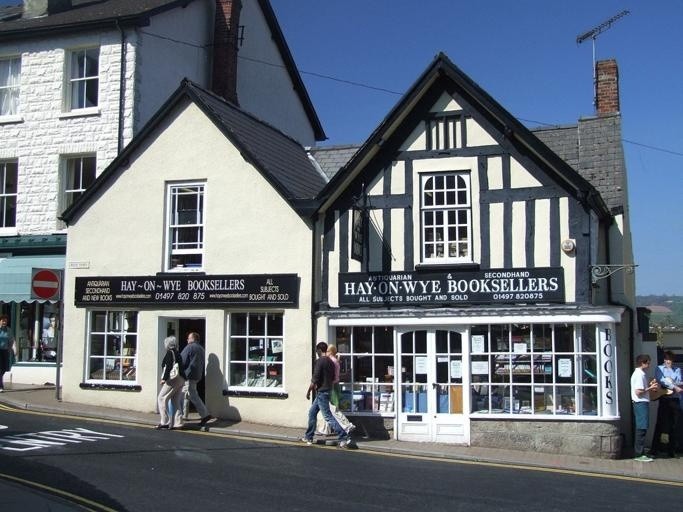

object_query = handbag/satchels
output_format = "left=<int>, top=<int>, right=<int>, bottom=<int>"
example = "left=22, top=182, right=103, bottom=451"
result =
left=161, top=362, right=180, bottom=383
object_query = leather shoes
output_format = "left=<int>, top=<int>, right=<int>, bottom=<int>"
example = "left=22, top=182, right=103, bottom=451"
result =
left=155, top=424, right=169, bottom=430
left=198, top=415, right=212, bottom=427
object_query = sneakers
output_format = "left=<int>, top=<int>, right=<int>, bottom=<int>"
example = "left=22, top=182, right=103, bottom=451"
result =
left=634, top=454, right=654, bottom=462
left=314, top=430, right=327, bottom=437
left=301, top=438, right=312, bottom=444
left=339, top=438, right=351, bottom=448
left=347, top=427, right=359, bottom=439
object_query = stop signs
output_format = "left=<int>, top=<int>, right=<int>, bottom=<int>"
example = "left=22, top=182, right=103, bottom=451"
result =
left=29, top=268, right=63, bottom=300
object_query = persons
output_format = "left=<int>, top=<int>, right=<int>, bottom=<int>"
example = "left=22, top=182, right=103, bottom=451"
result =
left=0, top=313, right=16, bottom=392
left=42, top=313, right=56, bottom=356
left=155, top=335, right=186, bottom=430
left=180, top=331, right=211, bottom=424
left=630, top=354, right=658, bottom=462
left=314, top=344, right=356, bottom=436
left=302, top=342, right=351, bottom=448
left=646, top=353, right=683, bottom=458
